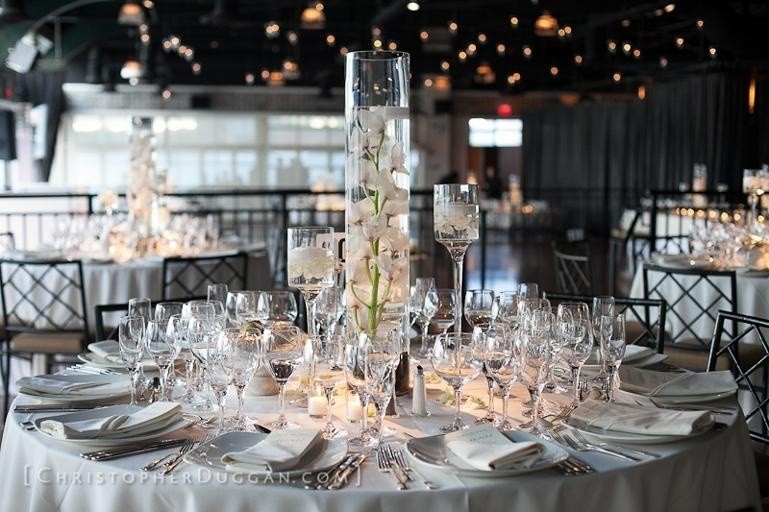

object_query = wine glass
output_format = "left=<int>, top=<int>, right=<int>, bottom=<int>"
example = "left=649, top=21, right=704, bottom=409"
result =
left=118, top=182, right=626, bottom=430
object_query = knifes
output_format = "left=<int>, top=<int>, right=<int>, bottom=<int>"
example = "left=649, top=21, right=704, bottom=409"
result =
left=80, top=437, right=192, bottom=463
left=303, top=450, right=369, bottom=492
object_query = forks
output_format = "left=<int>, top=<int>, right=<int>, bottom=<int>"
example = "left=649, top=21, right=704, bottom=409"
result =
left=373, top=445, right=440, bottom=494
left=137, top=429, right=219, bottom=478
left=547, top=425, right=664, bottom=464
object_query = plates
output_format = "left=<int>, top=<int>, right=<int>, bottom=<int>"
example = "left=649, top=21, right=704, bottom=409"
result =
left=565, top=399, right=715, bottom=443
left=32, top=403, right=202, bottom=445
left=78, top=348, right=189, bottom=370
left=579, top=346, right=669, bottom=373
left=405, top=433, right=573, bottom=476
left=607, top=369, right=739, bottom=401
left=657, top=256, right=714, bottom=270
left=18, top=374, right=139, bottom=399
left=188, top=431, right=351, bottom=477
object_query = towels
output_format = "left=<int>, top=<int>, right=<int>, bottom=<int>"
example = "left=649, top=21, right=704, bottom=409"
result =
left=569, top=397, right=717, bottom=437
left=221, top=428, right=324, bottom=476
left=442, top=422, right=548, bottom=471
left=15, top=364, right=112, bottom=394
left=615, top=362, right=739, bottom=396
left=579, top=344, right=656, bottom=364
left=40, top=400, right=183, bottom=439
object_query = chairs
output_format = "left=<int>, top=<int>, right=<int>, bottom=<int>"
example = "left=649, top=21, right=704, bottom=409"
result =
left=642, top=264, right=764, bottom=379
left=0, top=258, right=96, bottom=425
left=162, top=251, right=248, bottom=302
left=530, top=185, right=667, bottom=354
left=704, top=309, right=768, bottom=446
left=94, top=294, right=207, bottom=340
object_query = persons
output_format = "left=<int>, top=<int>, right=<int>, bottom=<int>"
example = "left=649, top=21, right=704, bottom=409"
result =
left=479, top=164, right=504, bottom=200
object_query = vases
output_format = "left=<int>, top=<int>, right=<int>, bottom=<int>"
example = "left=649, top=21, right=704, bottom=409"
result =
left=128, top=116, right=157, bottom=239
left=342, top=50, right=412, bottom=415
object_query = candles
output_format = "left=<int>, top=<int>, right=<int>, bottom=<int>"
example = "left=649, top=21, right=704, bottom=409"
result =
left=348, top=401, right=363, bottom=420
left=309, top=396, right=326, bottom=416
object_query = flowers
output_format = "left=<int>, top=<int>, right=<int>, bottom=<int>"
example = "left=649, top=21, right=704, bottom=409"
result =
left=345, top=106, right=412, bottom=344
left=130, top=132, right=156, bottom=208
left=433, top=206, right=480, bottom=241
left=288, top=246, right=338, bottom=280
